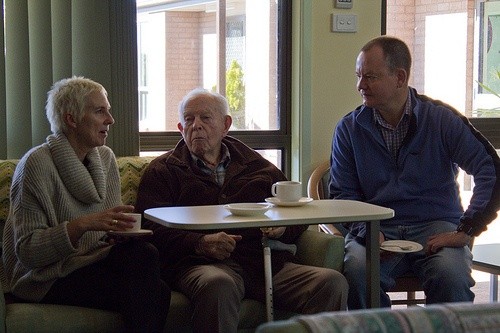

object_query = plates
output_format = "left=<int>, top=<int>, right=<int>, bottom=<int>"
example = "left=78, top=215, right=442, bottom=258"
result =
left=379, top=240, right=424, bottom=252
left=110, top=229, right=153, bottom=235
left=224, top=203, right=273, bottom=216
left=264, top=197, right=313, bottom=206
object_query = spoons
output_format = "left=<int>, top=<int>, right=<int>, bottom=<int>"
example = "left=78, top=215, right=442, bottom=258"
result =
left=381, top=245, right=413, bottom=250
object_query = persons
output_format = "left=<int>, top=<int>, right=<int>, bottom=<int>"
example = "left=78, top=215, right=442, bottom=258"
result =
left=133, top=87, right=349, bottom=333
left=328, top=36, right=500, bottom=312
left=2, top=75, right=173, bottom=333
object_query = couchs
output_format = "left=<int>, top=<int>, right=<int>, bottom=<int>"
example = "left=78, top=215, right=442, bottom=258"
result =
left=0, top=156, right=346, bottom=333
left=255, top=302, right=500, bottom=333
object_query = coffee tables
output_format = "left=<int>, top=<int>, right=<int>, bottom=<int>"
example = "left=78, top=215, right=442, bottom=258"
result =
left=144, top=200, right=394, bottom=325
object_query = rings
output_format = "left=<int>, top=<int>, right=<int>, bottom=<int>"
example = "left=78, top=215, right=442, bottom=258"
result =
left=112, top=220, right=118, bottom=225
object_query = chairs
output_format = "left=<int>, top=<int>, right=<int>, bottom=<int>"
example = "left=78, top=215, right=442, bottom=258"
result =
left=307, top=159, right=428, bottom=308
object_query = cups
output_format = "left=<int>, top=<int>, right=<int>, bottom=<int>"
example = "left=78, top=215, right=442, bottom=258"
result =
left=271, top=181, right=302, bottom=202
left=122, top=213, right=141, bottom=230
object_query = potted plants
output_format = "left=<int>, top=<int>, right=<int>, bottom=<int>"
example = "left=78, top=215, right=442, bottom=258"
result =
left=211, top=59, right=245, bottom=129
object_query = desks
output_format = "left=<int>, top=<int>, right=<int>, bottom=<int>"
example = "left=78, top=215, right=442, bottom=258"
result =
left=470, top=243, right=500, bottom=302
